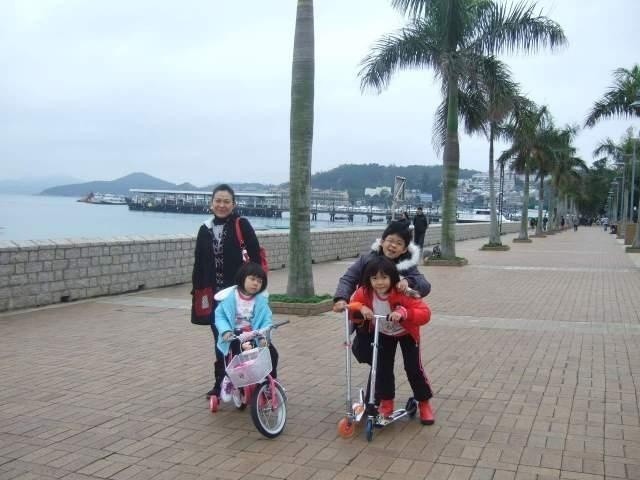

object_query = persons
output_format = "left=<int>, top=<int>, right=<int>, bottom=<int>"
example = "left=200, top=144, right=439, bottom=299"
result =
left=212, top=261, right=280, bottom=401
left=400, top=212, right=412, bottom=230
left=330, top=221, right=432, bottom=419
left=412, top=205, right=428, bottom=251
left=187, top=181, right=270, bottom=404
left=347, top=254, right=437, bottom=428
left=529, top=213, right=610, bottom=232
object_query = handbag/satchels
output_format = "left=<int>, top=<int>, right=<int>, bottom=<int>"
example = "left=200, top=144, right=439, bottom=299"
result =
left=242, top=246, right=268, bottom=273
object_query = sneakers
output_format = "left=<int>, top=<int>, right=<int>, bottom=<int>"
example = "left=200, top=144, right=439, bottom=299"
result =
left=207, top=383, right=221, bottom=398
left=220, top=375, right=233, bottom=403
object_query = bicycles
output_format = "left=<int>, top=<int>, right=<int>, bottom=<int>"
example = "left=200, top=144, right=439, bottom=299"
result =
left=208, top=318, right=291, bottom=439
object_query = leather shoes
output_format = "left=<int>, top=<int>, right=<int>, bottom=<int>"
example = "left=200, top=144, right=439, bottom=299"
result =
left=419, top=400, right=434, bottom=425
left=377, top=400, right=393, bottom=417
